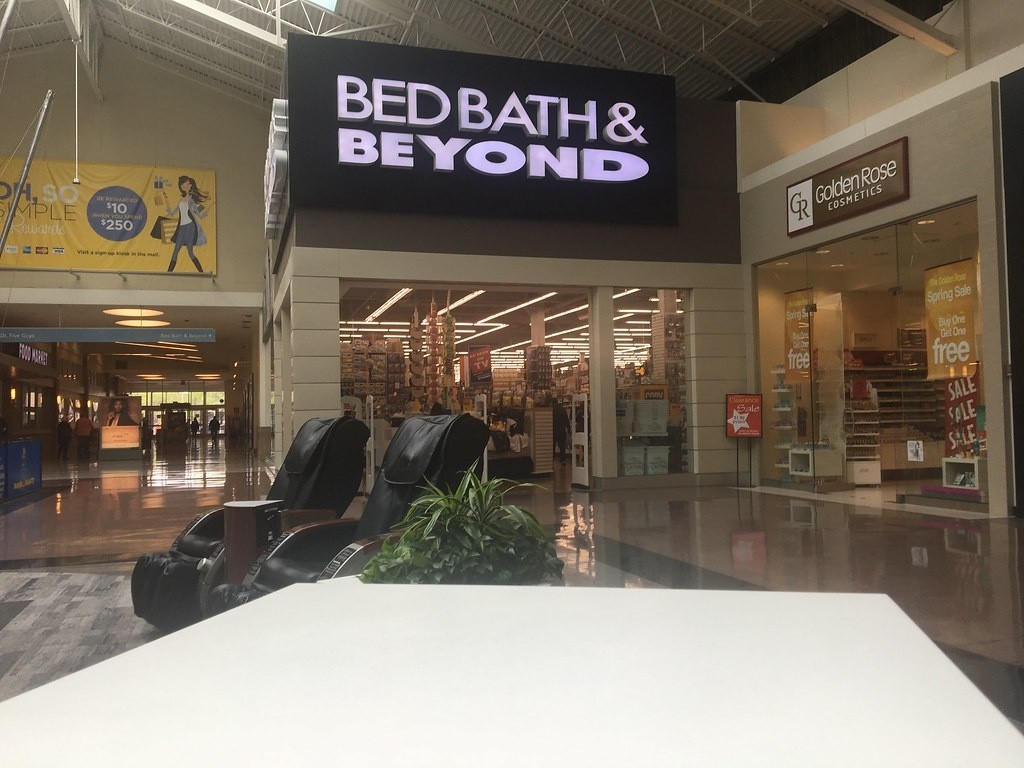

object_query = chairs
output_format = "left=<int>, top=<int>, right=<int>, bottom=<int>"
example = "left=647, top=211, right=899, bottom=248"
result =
left=229, top=413, right=490, bottom=612
left=131, top=416, right=370, bottom=634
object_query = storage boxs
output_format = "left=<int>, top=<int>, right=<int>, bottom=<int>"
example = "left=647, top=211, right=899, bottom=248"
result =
left=615, top=399, right=671, bottom=433
left=618, top=446, right=671, bottom=475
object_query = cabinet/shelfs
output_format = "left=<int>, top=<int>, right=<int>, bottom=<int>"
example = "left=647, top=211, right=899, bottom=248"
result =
left=815, top=365, right=946, bottom=489
left=771, top=369, right=796, bottom=468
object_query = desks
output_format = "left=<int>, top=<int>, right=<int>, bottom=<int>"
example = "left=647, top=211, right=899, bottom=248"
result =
left=617, top=433, right=670, bottom=436
left=2, top=583, right=1024, bottom=765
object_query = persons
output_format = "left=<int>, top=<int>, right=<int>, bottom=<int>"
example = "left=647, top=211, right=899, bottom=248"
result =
left=75, top=411, right=92, bottom=459
left=576, top=401, right=591, bottom=461
left=106, top=397, right=139, bottom=425
left=57, top=416, right=72, bottom=460
left=553, top=398, right=572, bottom=463
left=191, top=417, right=200, bottom=440
left=209, top=416, right=220, bottom=442
